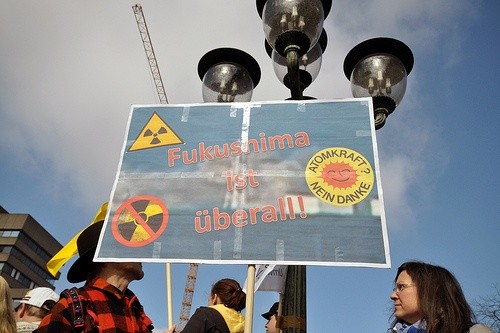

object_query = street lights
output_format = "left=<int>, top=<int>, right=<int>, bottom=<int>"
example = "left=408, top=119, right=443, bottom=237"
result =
left=199, top=0, right=414, bottom=333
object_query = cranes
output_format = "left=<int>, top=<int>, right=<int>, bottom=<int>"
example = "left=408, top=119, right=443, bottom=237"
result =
left=129, top=4, right=198, bottom=332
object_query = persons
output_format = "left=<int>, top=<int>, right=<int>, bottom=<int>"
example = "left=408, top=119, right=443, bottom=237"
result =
left=0, top=275, right=60, bottom=333
left=387, top=261, right=496, bottom=333
left=178, top=278, right=246, bottom=333
left=261, top=302, right=280, bottom=333
left=31, top=219, right=178, bottom=333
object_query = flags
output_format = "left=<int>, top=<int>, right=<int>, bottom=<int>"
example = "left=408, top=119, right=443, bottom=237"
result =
left=242, top=265, right=287, bottom=294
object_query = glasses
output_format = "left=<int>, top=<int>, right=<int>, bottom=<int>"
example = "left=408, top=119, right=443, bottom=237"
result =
left=393, top=282, right=415, bottom=293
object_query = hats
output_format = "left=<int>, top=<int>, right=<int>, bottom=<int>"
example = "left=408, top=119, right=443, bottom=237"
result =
left=67, top=220, right=104, bottom=283
left=261, top=302, right=279, bottom=321
left=20, top=287, right=61, bottom=310
